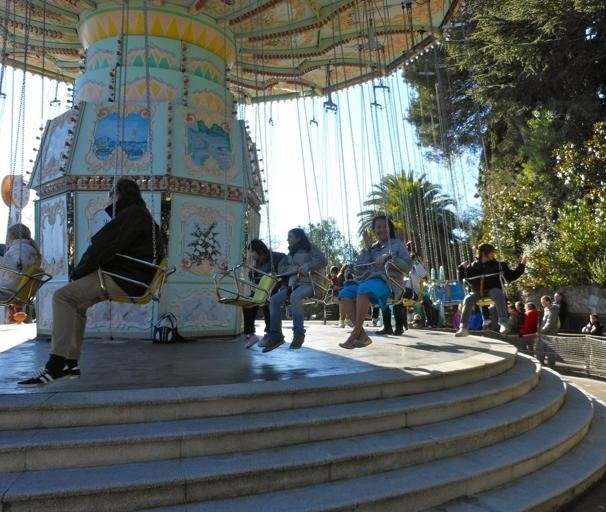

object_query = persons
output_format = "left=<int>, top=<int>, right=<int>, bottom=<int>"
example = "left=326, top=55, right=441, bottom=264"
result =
left=0, top=224, right=44, bottom=303
left=241, top=238, right=287, bottom=348
left=581, top=312, right=601, bottom=377
left=452, top=243, right=530, bottom=339
left=15, top=177, right=168, bottom=387
left=454, top=290, right=567, bottom=371
left=321, top=253, right=432, bottom=336
left=338, top=215, right=415, bottom=350
left=260, top=227, right=330, bottom=355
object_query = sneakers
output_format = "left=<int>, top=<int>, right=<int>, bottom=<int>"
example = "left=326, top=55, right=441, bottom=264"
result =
left=339, top=335, right=373, bottom=349
left=289, top=333, right=306, bottom=350
left=16, top=365, right=81, bottom=388
left=338, top=319, right=354, bottom=328
left=243, top=334, right=258, bottom=349
left=375, top=327, right=393, bottom=335
left=500, top=324, right=512, bottom=334
left=455, top=327, right=470, bottom=337
left=393, top=329, right=404, bottom=336
left=372, top=318, right=378, bottom=327
left=258, top=333, right=286, bottom=353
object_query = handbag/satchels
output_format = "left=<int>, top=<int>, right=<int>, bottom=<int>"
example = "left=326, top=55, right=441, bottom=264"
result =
left=152, top=311, right=179, bottom=344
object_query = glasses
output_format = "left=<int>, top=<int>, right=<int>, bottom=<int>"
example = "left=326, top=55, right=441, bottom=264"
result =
left=108, top=190, right=121, bottom=197
